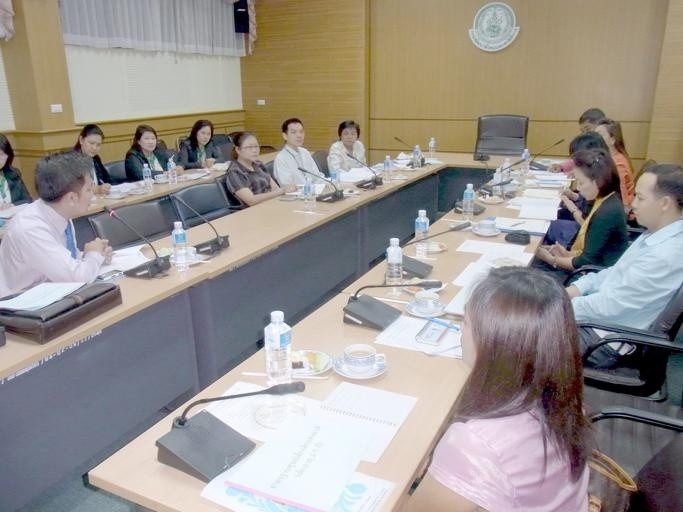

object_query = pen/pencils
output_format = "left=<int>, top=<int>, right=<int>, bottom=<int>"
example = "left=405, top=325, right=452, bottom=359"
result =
left=511, top=222, right=525, bottom=227
left=427, top=315, right=461, bottom=332
left=99, top=177, right=104, bottom=185
left=559, top=186, right=567, bottom=196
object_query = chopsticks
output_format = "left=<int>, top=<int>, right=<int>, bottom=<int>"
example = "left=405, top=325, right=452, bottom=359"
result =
left=293, top=210, right=325, bottom=218
left=240, top=371, right=331, bottom=381
left=350, top=294, right=410, bottom=306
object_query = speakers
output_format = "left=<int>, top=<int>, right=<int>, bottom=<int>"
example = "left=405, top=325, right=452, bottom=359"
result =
left=232, top=1, right=251, bottom=34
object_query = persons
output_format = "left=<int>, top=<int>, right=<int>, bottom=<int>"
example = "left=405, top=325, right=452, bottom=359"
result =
left=388, top=265, right=592, bottom=510
left=536, top=109, right=635, bottom=285
left=225, top=131, right=299, bottom=206
left=325, top=121, right=366, bottom=179
left=72, top=123, right=116, bottom=197
left=272, top=118, right=334, bottom=190
left=0, top=133, right=33, bottom=213
left=124, top=124, right=185, bottom=182
left=563, top=163, right=682, bottom=371
left=177, top=120, right=226, bottom=170
left=0, top=150, right=115, bottom=301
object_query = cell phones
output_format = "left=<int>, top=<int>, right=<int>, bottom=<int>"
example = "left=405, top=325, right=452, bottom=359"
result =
left=98, top=268, right=124, bottom=284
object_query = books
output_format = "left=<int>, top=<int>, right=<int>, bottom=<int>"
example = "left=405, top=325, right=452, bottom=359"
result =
left=494, top=216, right=551, bottom=236
left=414, top=316, right=452, bottom=346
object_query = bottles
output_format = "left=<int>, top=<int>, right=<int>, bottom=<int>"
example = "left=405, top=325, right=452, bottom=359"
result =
left=462, top=184, right=475, bottom=222
left=383, top=155, right=392, bottom=179
left=385, top=238, right=404, bottom=288
left=521, top=149, right=530, bottom=173
left=414, top=209, right=429, bottom=260
left=428, top=138, right=438, bottom=161
left=330, top=166, right=340, bottom=189
left=142, top=163, right=153, bottom=193
left=171, top=220, right=191, bottom=273
left=412, top=145, right=422, bottom=168
left=166, top=158, right=178, bottom=186
left=303, top=178, right=316, bottom=211
left=493, top=168, right=504, bottom=201
left=502, top=158, right=511, bottom=176
left=263, top=309, right=293, bottom=388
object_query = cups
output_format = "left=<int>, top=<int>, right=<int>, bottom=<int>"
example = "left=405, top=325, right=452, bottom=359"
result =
left=414, top=290, right=440, bottom=314
left=345, top=343, right=387, bottom=374
left=473, top=221, right=496, bottom=233
left=172, top=246, right=197, bottom=260
left=155, top=174, right=165, bottom=181
left=109, top=188, right=123, bottom=196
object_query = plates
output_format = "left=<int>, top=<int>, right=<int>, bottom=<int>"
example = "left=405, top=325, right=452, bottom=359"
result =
left=479, top=196, right=504, bottom=205
left=333, top=358, right=389, bottom=381
left=169, top=256, right=202, bottom=265
left=401, top=278, right=446, bottom=295
left=413, top=241, right=446, bottom=253
left=471, top=229, right=501, bottom=237
left=389, top=175, right=409, bottom=180
left=405, top=302, right=449, bottom=319
left=104, top=194, right=125, bottom=199
left=288, top=349, right=334, bottom=375
left=151, top=179, right=170, bottom=184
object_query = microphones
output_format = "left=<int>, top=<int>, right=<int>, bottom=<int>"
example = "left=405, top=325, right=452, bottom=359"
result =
left=156, top=379, right=305, bottom=484
left=100, top=204, right=171, bottom=279
left=342, top=279, right=443, bottom=331
left=346, top=152, right=382, bottom=189
left=502, top=157, right=526, bottom=171
left=394, top=136, right=425, bottom=167
left=298, top=166, right=344, bottom=203
left=401, top=222, right=473, bottom=279
left=530, top=138, right=567, bottom=172
left=169, top=192, right=230, bottom=254
left=455, top=178, right=515, bottom=215
